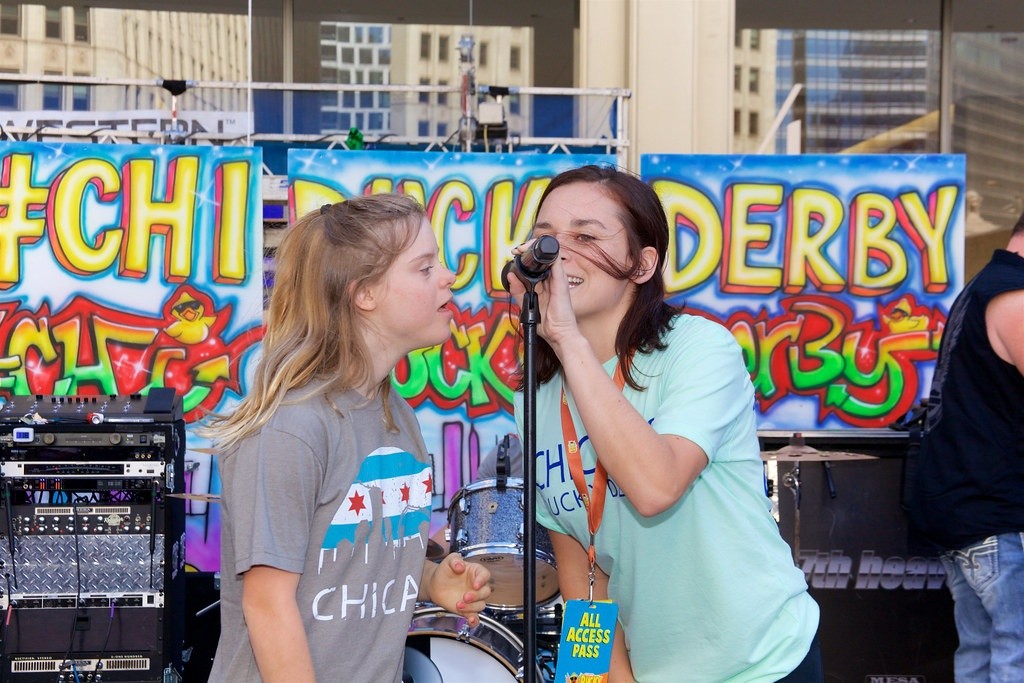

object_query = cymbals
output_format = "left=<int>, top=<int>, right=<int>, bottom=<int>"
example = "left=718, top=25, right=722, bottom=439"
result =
left=761, top=443, right=880, bottom=462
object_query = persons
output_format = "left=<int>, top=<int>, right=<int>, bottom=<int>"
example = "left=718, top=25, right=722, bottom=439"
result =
left=195, top=193, right=491, bottom=683
left=426, top=434, right=524, bottom=558
left=922, top=211, right=1024, bottom=683
left=507, top=165, right=824, bottom=683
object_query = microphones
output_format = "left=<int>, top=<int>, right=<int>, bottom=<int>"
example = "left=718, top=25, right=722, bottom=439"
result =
left=499, top=232, right=559, bottom=292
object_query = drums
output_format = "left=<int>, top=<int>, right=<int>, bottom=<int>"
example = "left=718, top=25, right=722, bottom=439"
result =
left=404, top=606, right=546, bottom=682
left=446, top=476, right=561, bottom=611
